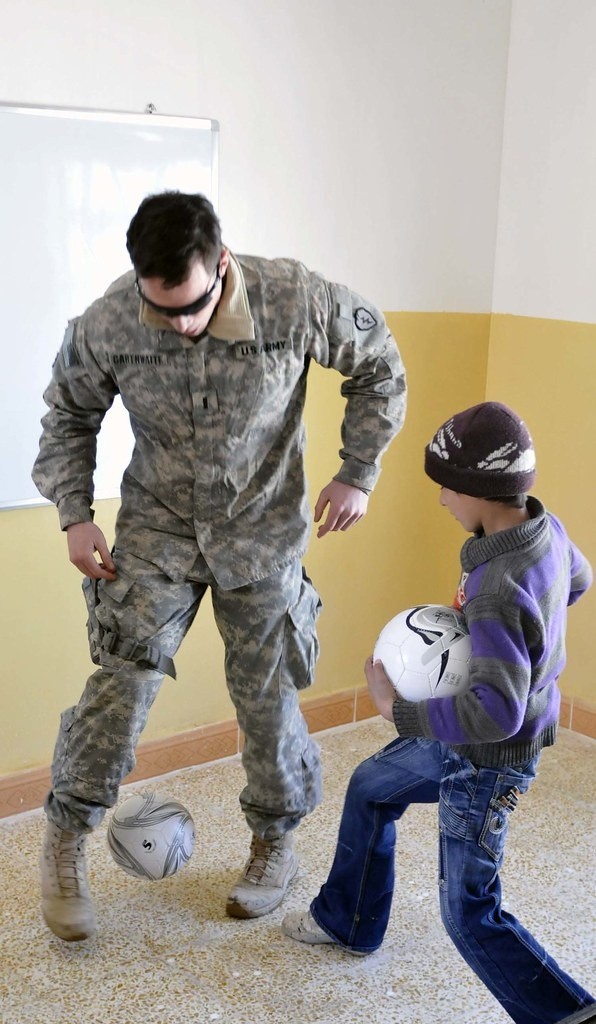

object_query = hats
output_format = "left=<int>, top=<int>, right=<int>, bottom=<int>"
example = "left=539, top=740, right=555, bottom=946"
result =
left=422, top=401, right=536, bottom=498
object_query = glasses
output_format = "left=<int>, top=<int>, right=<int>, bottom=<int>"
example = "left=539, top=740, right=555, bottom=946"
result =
left=134, top=261, right=220, bottom=318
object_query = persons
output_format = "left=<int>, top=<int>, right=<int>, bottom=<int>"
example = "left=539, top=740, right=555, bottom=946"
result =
left=30, top=189, right=407, bottom=941
left=280, top=401, right=595, bottom=1023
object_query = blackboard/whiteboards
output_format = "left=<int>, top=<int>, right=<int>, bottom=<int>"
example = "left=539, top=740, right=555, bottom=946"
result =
left=0, top=100, right=220, bottom=512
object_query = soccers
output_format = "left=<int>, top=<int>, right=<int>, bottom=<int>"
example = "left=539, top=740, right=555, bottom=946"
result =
left=107, top=792, right=196, bottom=881
left=373, top=603, right=473, bottom=703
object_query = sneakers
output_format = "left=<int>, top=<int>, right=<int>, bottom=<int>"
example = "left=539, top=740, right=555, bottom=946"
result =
left=281, top=908, right=381, bottom=957
left=224, top=831, right=298, bottom=918
left=556, top=1002, right=596, bottom=1024
left=38, top=819, right=97, bottom=943
